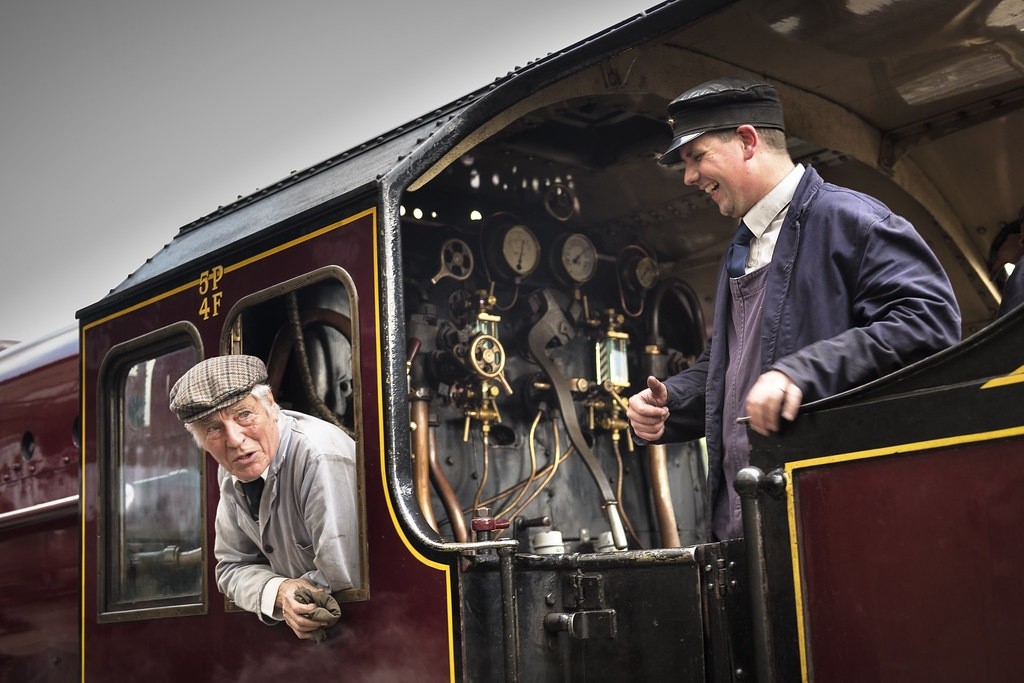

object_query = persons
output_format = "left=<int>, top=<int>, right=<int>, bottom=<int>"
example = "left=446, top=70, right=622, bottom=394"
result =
left=627, top=78, right=962, bottom=546
left=168, top=354, right=362, bottom=640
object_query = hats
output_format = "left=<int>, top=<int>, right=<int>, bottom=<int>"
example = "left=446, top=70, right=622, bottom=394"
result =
left=168, top=355, right=269, bottom=424
left=658, top=78, right=785, bottom=165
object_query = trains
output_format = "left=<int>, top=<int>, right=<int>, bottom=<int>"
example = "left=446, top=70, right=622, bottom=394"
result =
left=0, top=0, right=1024, bottom=683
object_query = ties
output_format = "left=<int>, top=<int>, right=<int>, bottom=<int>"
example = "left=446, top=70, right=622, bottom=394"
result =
left=242, top=477, right=266, bottom=518
left=728, top=224, right=753, bottom=279
